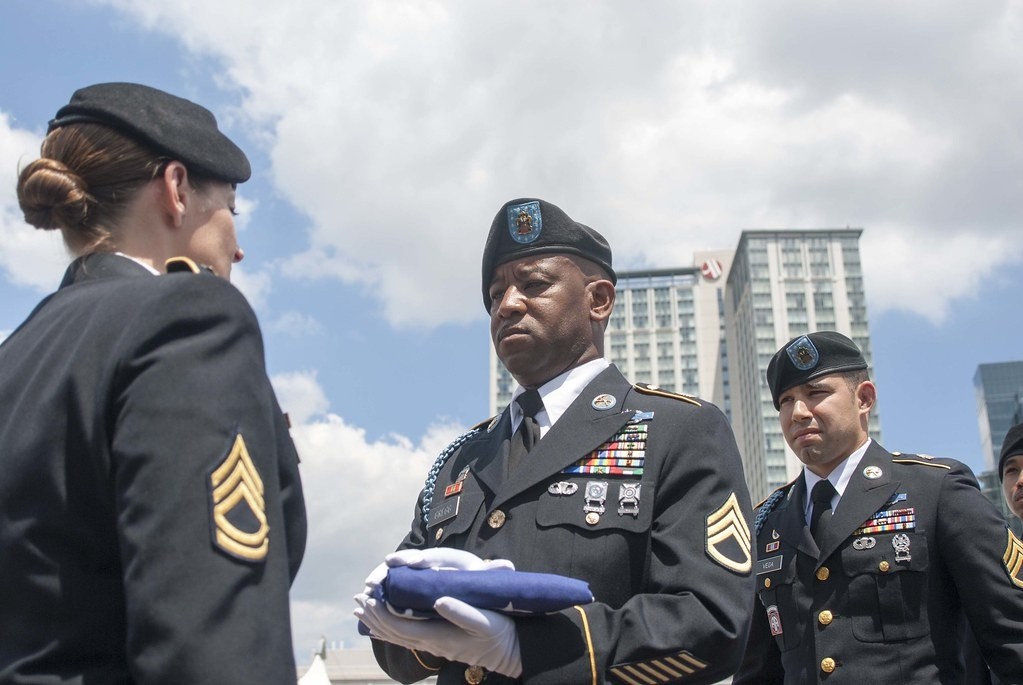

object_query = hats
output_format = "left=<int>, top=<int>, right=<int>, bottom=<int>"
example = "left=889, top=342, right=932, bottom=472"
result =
left=482, top=198, right=618, bottom=321
left=998, top=423, right=1023, bottom=484
left=767, top=332, right=868, bottom=411
left=48, top=82, right=252, bottom=183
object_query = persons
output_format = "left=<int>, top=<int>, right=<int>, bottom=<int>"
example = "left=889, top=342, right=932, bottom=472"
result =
left=353, top=197, right=756, bottom=685
left=997, top=423, right=1023, bottom=517
left=732, top=332, right=1023, bottom=685
left=0, top=82, right=307, bottom=685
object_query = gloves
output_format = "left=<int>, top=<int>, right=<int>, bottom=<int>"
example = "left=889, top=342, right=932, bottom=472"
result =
left=351, top=594, right=523, bottom=679
left=362, top=547, right=516, bottom=595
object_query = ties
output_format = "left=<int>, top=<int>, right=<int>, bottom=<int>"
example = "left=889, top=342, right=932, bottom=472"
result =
left=507, top=391, right=544, bottom=482
left=810, top=480, right=837, bottom=551
